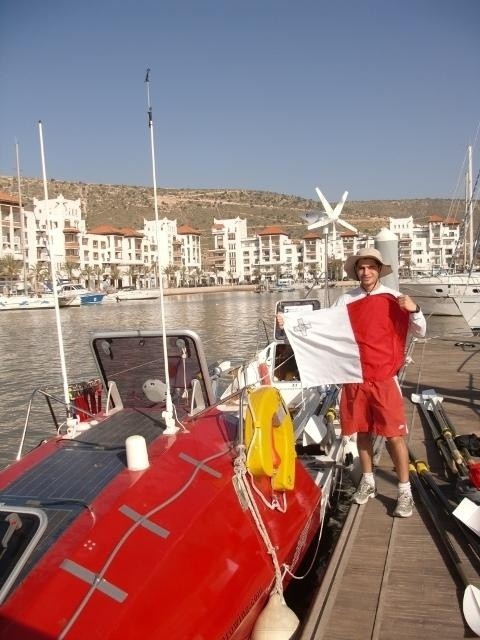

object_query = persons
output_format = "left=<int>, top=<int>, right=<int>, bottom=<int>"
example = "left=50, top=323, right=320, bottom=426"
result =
left=276, top=247, right=426, bottom=518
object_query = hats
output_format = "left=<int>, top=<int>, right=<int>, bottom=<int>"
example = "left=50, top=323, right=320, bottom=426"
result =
left=344, top=246, right=394, bottom=281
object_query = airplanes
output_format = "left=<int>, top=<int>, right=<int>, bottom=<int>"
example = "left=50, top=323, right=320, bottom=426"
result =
left=0, top=180, right=359, bottom=640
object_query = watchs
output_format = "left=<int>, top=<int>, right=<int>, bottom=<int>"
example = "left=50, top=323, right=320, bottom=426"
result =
left=410, top=305, right=420, bottom=313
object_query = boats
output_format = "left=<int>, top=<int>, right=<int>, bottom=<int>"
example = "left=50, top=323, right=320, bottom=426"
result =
left=267, top=278, right=336, bottom=292
left=0, top=143, right=162, bottom=310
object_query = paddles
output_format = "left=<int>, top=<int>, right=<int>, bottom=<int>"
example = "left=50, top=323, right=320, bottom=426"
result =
left=384, top=389, right=480, bottom=636
left=303, top=383, right=341, bottom=456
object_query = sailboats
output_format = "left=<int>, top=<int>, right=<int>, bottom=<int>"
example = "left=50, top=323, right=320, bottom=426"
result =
left=394, top=139, right=479, bottom=330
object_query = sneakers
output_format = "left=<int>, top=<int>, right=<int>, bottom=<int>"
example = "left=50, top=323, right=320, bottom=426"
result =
left=391, top=492, right=416, bottom=518
left=349, top=479, right=377, bottom=506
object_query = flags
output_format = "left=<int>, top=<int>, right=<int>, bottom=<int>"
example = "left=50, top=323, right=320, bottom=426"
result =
left=278, top=293, right=410, bottom=390
